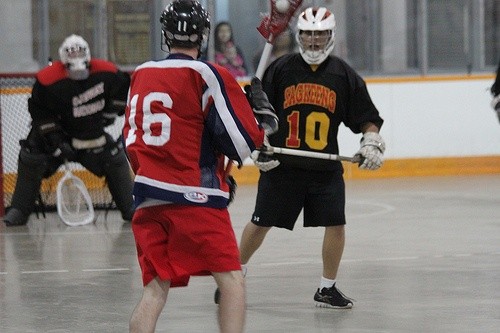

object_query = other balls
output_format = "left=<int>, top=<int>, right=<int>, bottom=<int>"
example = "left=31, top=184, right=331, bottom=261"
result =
left=275, top=0, right=289, bottom=13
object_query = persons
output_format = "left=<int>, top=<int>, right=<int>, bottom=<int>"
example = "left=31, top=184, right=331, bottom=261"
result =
left=120, top=0, right=278, bottom=333
left=215, top=7, right=386, bottom=310
left=2, top=34, right=135, bottom=226
left=214, top=22, right=248, bottom=78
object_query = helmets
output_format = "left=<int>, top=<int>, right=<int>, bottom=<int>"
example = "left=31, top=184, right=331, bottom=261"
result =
left=58, top=33, right=90, bottom=71
left=160, top=0, right=210, bottom=46
left=295, top=6, right=336, bottom=65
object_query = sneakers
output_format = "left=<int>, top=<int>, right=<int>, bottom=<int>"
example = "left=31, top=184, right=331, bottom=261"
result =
left=214, top=288, right=221, bottom=304
left=313, top=282, right=357, bottom=310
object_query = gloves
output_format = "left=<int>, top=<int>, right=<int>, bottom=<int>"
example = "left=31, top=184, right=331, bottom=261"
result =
left=249, top=135, right=280, bottom=172
left=244, top=78, right=277, bottom=119
left=92, top=134, right=119, bottom=156
left=351, top=131, right=386, bottom=171
left=38, top=128, right=68, bottom=164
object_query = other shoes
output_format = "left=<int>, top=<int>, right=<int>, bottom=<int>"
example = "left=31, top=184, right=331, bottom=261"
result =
left=121, top=201, right=136, bottom=221
left=3, top=206, right=28, bottom=226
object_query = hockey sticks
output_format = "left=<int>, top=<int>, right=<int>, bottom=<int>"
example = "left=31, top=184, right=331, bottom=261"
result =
left=223, top=0, right=303, bottom=183
left=254, top=144, right=353, bottom=162
left=56, top=158, right=95, bottom=226
